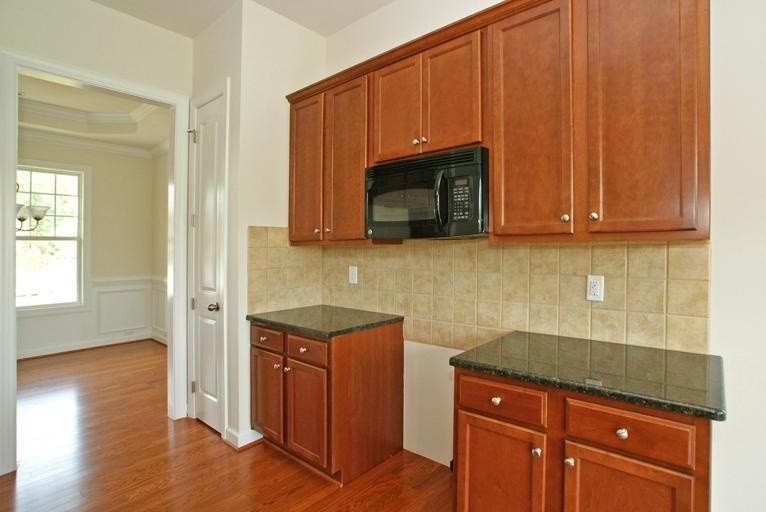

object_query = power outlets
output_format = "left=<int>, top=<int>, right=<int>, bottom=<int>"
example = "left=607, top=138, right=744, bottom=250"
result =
left=587, top=275, right=604, bottom=302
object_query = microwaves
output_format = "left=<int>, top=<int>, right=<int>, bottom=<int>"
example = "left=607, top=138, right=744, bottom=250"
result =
left=365, top=146, right=489, bottom=239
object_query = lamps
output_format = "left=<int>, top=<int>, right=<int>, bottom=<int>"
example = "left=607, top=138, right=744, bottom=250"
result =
left=17, top=183, right=50, bottom=231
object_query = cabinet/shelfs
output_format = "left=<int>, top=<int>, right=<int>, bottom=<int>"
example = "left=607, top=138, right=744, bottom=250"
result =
left=453, top=366, right=710, bottom=512
left=483, top=0, right=710, bottom=245
left=368, top=10, right=483, bottom=165
left=286, top=60, right=368, bottom=245
left=250, top=321, right=403, bottom=489
